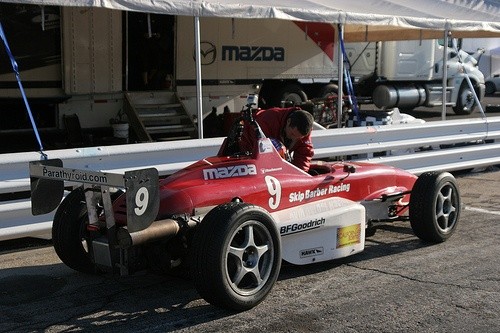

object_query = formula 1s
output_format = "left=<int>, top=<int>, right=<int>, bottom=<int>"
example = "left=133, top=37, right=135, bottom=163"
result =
left=29, top=103, right=461, bottom=309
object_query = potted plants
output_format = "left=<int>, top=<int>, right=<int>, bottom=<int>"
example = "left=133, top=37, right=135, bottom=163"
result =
left=108, top=109, right=130, bottom=138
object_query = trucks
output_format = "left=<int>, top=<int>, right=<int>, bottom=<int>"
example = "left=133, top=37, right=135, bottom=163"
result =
left=61, top=7, right=485, bottom=138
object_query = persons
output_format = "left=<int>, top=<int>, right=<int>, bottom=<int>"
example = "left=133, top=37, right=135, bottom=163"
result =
left=218, top=106, right=315, bottom=172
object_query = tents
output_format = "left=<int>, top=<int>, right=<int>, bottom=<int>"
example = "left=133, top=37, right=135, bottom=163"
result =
left=1, top=0, right=500, bottom=139
